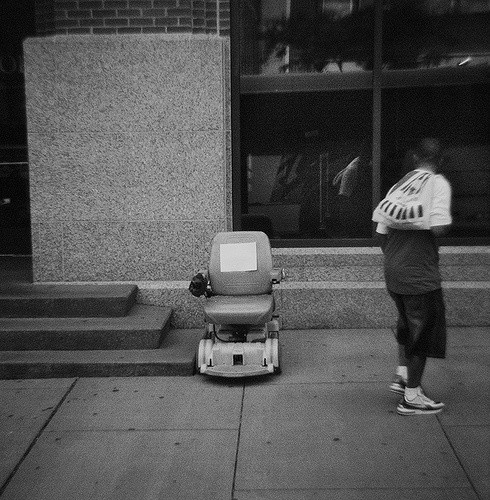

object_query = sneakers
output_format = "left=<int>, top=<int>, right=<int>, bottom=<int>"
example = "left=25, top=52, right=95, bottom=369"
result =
left=396, top=394, right=445, bottom=416
left=389, top=375, right=426, bottom=396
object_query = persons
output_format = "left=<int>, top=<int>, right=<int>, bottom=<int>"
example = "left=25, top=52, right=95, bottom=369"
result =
left=371, top=135, right=452, bottom=415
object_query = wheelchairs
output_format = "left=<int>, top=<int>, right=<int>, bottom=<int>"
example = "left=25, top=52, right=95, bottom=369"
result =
left=188, top=231, right=282, bottom=377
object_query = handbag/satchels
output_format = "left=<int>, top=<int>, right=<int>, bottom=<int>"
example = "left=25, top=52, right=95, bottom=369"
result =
left=332, top=168, right=346, bottom=185
left=371, top=171, right=435, bottom=231
